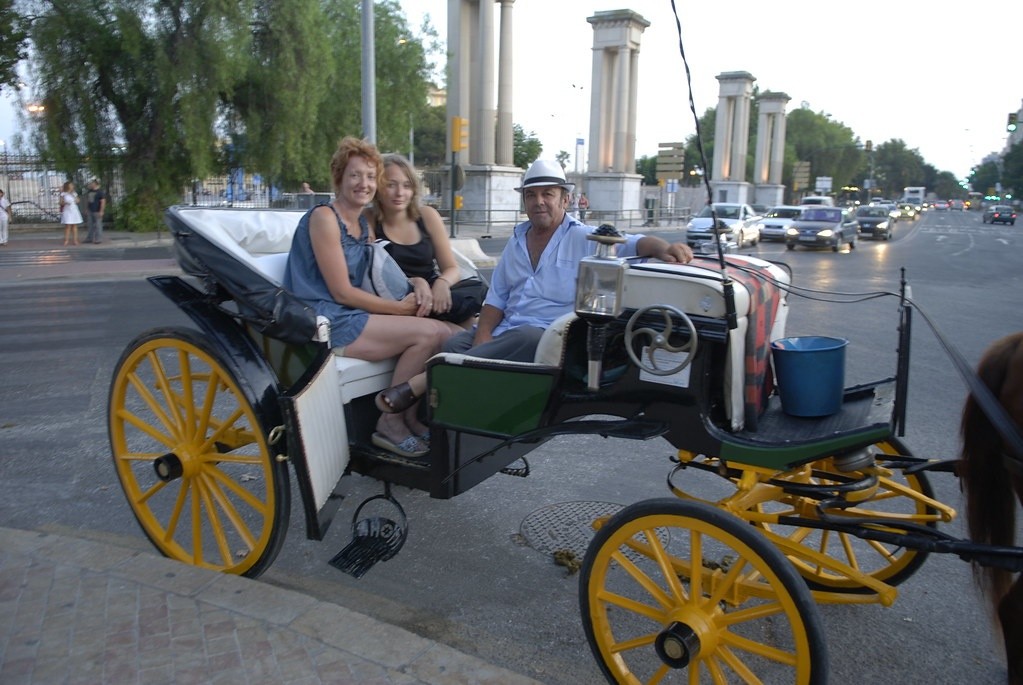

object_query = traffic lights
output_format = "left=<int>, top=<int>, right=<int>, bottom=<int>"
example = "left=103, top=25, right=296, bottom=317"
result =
left=451, top=116, right=469, bottom=152
left=455, top=195, right=463, bottom=208
left=1007, top=113, right=1017, bottom=132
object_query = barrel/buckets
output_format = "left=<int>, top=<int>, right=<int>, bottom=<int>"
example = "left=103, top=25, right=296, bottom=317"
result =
left=773, top=335, right=849, bottom=419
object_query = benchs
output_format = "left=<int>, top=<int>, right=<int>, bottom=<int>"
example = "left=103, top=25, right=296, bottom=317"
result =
left=166, top=204, right=488, bottom=399
left=426, top=310, right=582, bottom=437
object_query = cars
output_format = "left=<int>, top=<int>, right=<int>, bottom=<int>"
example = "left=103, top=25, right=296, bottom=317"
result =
left=686, top=202, right=760, bottom=251
left=760, top=196, right=923, bottom=252
left=923, top=199, right=964, bottom=211
left=983, top=205, right=1017, bottom=226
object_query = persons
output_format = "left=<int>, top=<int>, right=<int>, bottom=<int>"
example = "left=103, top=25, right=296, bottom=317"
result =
left=283, top=136, right=453, bottom=457
left=60, top=182, right=83, bottom=246
left=81, top=176, right=106, bottom=243
left=578, top=192, right=589, bottom=224
left=0, top=189, right=11, bottom=246
left=360, top=155, right=490, bottom=335
left=303, top=183, right=313, bottom=193
left=375, top=156, right=693, bottom=414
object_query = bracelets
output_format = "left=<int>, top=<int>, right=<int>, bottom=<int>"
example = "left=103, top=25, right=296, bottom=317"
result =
left=438, top=277, right=450, bottom=285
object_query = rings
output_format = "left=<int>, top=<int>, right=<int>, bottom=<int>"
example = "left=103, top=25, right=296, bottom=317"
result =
left=429, top=304, right=433, bottom=306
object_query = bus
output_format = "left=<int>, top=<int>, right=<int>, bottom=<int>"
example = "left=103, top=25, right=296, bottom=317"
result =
left=904, top=187, right=926, bottom=208
left=967, top=192, right=983, bottom=209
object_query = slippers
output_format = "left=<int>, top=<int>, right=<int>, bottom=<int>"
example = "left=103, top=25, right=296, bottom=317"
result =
left=372, top=432, right=430, bottom=457
left=412, top=428, right=433, bottom=447
left=374, top=382, right=427, bottom=414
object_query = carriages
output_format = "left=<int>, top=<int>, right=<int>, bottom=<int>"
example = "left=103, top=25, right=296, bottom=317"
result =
left=106, top=204, right=1023, bottom=685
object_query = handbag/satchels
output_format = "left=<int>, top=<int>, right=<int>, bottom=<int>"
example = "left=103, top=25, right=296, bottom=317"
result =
left=424, top=278, right=488, bottom=324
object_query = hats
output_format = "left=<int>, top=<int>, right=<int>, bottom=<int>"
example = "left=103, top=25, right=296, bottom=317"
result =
left=513, top=158, right=575, bottom=193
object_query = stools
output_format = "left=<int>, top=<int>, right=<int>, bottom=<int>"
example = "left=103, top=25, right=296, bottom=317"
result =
left=619, top=248, right=792, bottom=431
